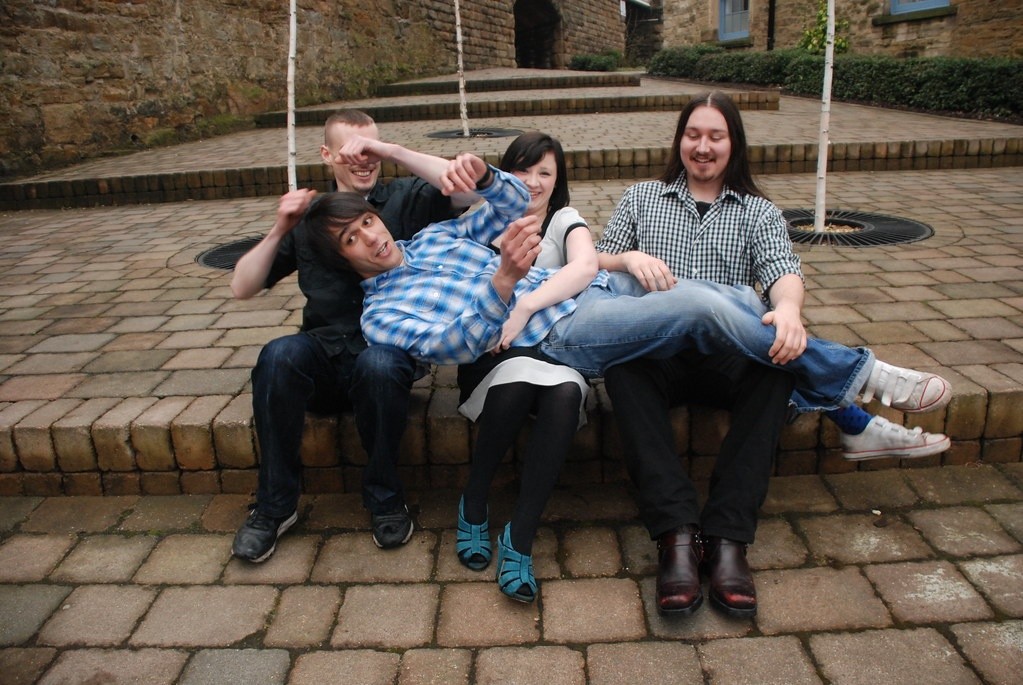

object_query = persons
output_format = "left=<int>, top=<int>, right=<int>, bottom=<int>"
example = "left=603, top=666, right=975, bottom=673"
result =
left=230, top=110, right=481, bottom=563
left=304, top=153, right=952, bottom=461
left=457, top=132, right=599, bottom=605
left=594, top=91, right=807, bottom=617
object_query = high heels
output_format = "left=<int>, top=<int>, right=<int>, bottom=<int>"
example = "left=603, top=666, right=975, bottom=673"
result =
left=456, top=491, right=494, bottom=571
left=494, top=522, right=537, bottom=603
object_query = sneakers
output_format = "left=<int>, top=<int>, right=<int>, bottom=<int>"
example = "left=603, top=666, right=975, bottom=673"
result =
left=839, top=414, right=951, bottom=462
left=231, top=503, right=296, bottom=562
left=858, top=359, right=953, bottom=413
left=372, top=503, right=414, bottom=550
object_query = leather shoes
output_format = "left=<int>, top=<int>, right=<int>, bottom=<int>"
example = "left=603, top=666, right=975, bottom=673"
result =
left=656, top=523, right=703, bottom=617
left=697, top=529, right=757, bottom=617
